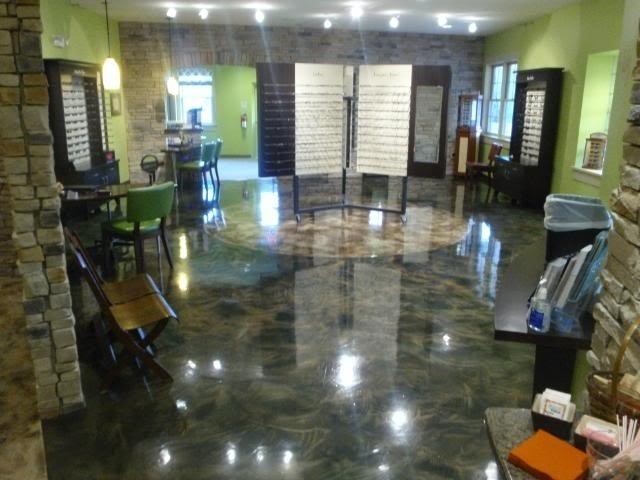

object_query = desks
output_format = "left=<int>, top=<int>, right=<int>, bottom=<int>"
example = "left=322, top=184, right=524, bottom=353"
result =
left=494, top=239, right=593, bottom=404
left=63, top=183, right=151, bottom=263
left=482, top=407, right=590, bottom=479
left=161, top=143, right=203, bottom=189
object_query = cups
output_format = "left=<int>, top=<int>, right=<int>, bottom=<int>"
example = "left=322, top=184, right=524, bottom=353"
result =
left=585, top=431, right=640, bottom=480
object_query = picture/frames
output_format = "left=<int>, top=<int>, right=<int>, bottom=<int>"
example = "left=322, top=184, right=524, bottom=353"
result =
left=110, top=93, right=122, bottom=116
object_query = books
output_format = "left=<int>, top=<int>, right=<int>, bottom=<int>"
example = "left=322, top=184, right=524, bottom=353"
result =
left=506, top=427, right=594, bottom=480
left=526, top=228, right=608, bottom=320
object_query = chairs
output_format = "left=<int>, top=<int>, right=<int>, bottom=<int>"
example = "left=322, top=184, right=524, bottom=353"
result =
left=175, top=141, right=215, bottom=192
left=464, top=142, right=502, bottom=190
left=100, top=180, right=174, bottom=277
left=191, top=139, right=223, bottom=188
left=62, top=225, right=181, bottom=394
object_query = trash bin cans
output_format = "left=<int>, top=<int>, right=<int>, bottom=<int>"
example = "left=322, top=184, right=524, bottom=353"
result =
left=544, top=194, right=611, bottom=269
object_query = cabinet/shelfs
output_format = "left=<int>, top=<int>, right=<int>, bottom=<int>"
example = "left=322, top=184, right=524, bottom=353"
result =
left=45, top=59, right=121, bottom=221
left=493, top=66, right=565, bottom=203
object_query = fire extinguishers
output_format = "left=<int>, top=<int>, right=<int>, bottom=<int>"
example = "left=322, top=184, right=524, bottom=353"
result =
left=241, top=113, right=247, bottom=128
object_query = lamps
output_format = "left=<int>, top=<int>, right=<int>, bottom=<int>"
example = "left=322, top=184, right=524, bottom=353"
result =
left=166, top=18, right=178, bottom=97
left=102, top=0, right=121, bottom=90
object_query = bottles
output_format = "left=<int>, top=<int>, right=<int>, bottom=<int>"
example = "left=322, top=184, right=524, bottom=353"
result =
left=528, top=278, right=551, bottom=333
